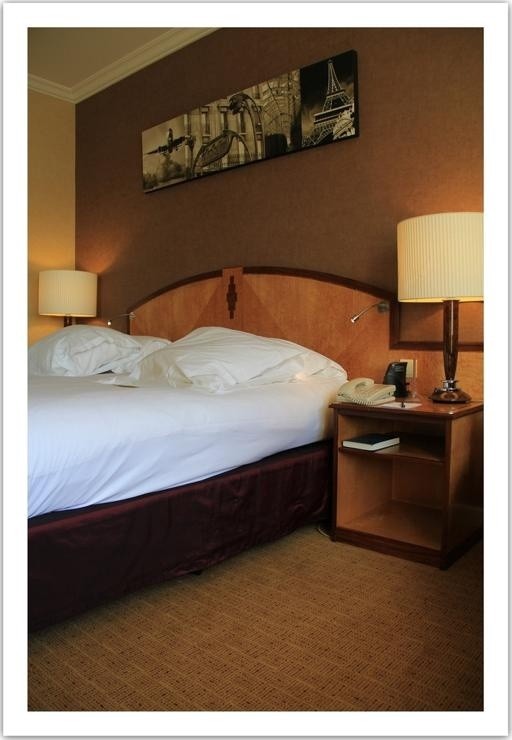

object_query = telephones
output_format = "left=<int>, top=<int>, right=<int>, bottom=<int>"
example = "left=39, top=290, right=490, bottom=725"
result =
left=336, top=377, right=396, bottom=405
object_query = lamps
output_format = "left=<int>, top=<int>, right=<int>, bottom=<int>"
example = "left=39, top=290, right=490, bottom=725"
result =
left=396, top=211, right=484, bottom=406
left=107, top=313, right=136, bottom=326
left=350, top=301, right=389, bottom=325
left=39, top=270, right=98, bottom=327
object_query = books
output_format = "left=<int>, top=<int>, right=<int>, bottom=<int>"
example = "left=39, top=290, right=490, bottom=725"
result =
left=343, top=433, right=400, bottom=451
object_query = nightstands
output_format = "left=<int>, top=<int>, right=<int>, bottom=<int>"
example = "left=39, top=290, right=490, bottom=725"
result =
left=329, top=397, right=484, bottom=571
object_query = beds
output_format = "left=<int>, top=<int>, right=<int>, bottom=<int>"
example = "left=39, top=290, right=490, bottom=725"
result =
left=27, top=267, right=399, bottom=634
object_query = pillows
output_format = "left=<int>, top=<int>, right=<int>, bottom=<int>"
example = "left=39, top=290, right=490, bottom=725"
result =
left=28, top=324, right=172, bottom=376
left=100, top=325, right=348, bottom=391
